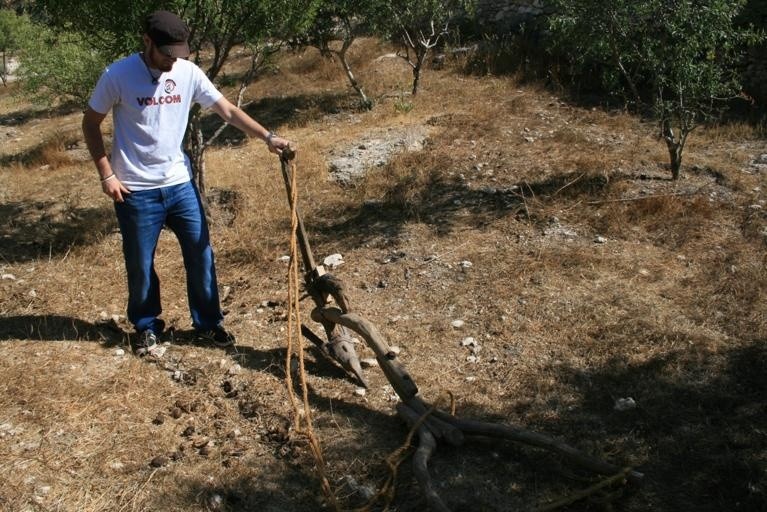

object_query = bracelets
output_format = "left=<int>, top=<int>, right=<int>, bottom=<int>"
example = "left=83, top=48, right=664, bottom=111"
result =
left=98, top=173, right=115, bottom=182
left=264, top=128, right=274, bottom=142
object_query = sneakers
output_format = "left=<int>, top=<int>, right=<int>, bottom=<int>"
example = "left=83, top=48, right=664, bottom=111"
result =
left=136, top=331, right=160, bottom=353
left=200, top=324, right=236, bottom=346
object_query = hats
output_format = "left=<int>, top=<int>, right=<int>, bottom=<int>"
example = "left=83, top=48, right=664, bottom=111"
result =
left=143, top=11, right=190, bottom=58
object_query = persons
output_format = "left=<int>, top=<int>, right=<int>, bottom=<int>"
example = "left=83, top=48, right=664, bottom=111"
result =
left=82, top=10, right=297, bottom=354
